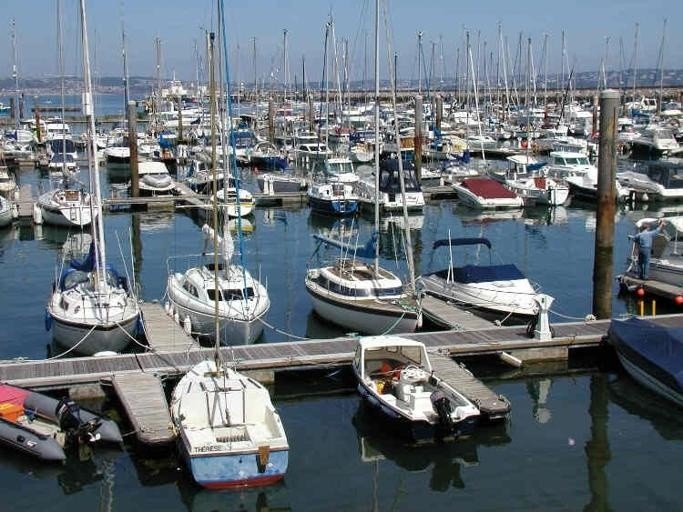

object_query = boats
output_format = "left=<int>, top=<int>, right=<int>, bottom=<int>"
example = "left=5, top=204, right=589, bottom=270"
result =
left=604, top=376, right=683, bottom=441
left=1, top=377, right=125, bottom=468
left=603, top=310, right=683, bottom=407
left=346, top=330, right=482, bottom=449
left=349, top=398, right=480, bottom=492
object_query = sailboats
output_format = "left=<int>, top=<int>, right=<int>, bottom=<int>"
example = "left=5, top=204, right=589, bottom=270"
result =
left=166, top=20, right=291, bottom=493
left=170, top=467, right=292, bottom=511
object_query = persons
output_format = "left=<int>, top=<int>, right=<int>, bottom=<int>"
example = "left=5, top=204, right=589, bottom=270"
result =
left=626, top=219, right=664, bottom=281
left=429, top=389, right=461, bottom=441
